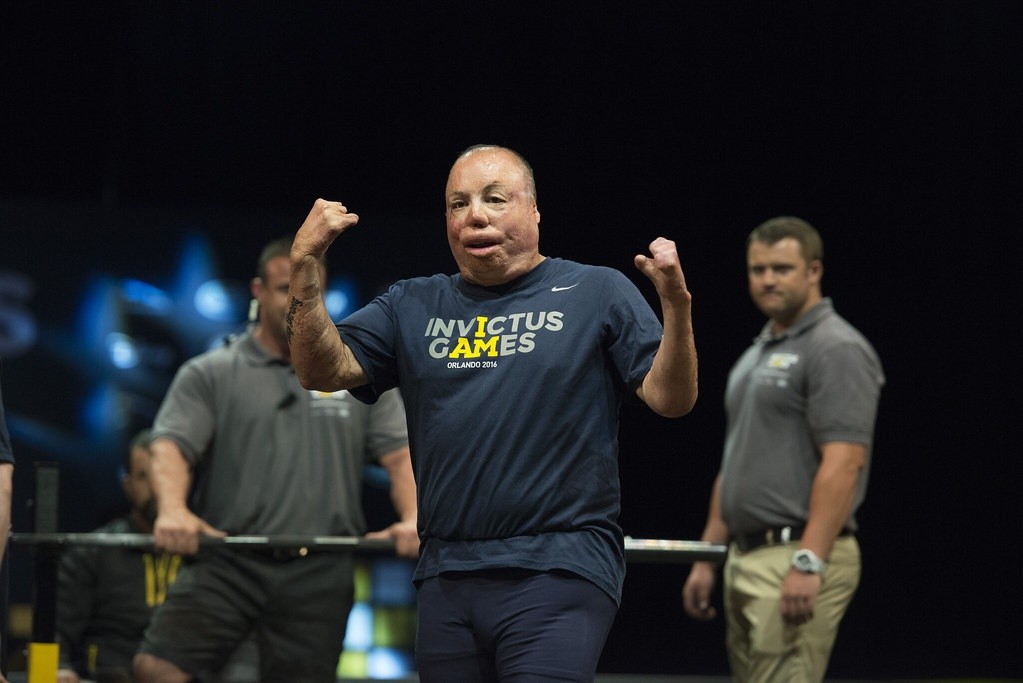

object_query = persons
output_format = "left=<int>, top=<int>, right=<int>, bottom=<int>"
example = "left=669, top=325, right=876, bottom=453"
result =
left=682, top=215, right=884, bottom=683
left=282, top=144, right=700, bottom=683
left=58, top=430, right=186, bottom=683
left=131, top=238, right=422, bottom=683
left=0, top=394, right=16, bottom=566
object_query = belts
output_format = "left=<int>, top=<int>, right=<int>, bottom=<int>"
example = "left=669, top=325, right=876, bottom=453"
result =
left=730, top=523, right=851, bottom=553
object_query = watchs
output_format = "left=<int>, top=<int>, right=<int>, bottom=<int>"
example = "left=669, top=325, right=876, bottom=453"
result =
left=790, top=548, right=828, bottom=574
